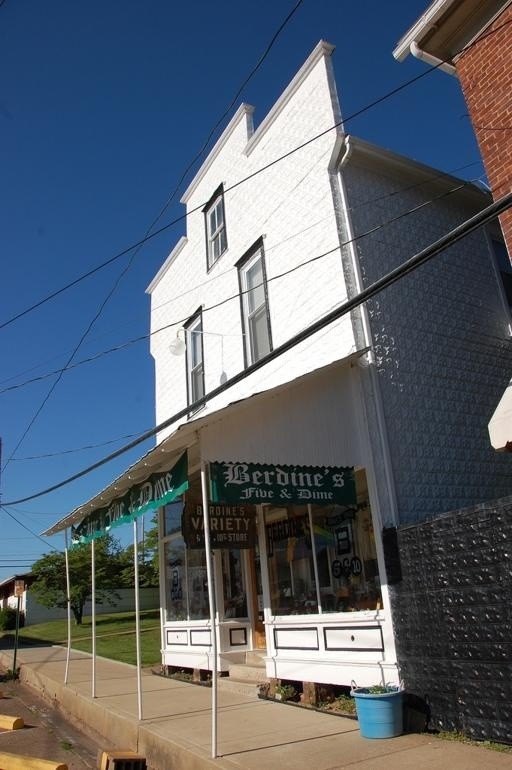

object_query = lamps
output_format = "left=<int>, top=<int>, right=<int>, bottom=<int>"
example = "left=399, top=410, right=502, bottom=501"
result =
left=168, top=327, right=225, bottom=357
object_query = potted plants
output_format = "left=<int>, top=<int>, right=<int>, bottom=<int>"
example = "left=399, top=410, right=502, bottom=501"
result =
left=349, top=679, right=406, bottom=739
